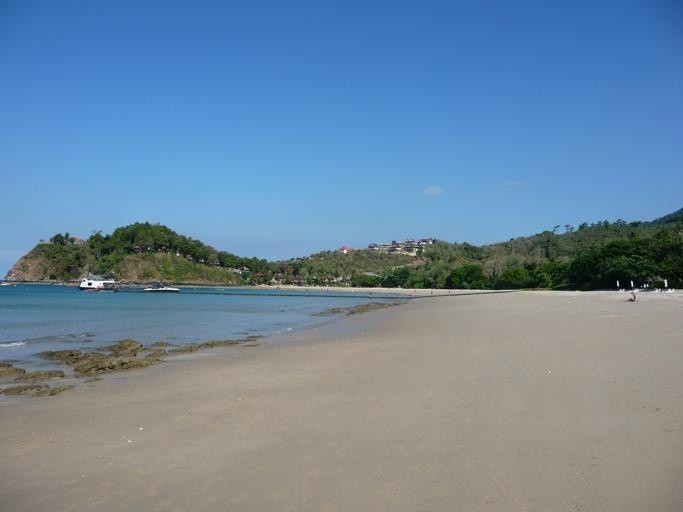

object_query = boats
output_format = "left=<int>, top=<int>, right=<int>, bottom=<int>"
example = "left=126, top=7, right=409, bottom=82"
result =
left=142, top=281, right=180, bottom=293
left=77, top=274, right=117, bottom=290
